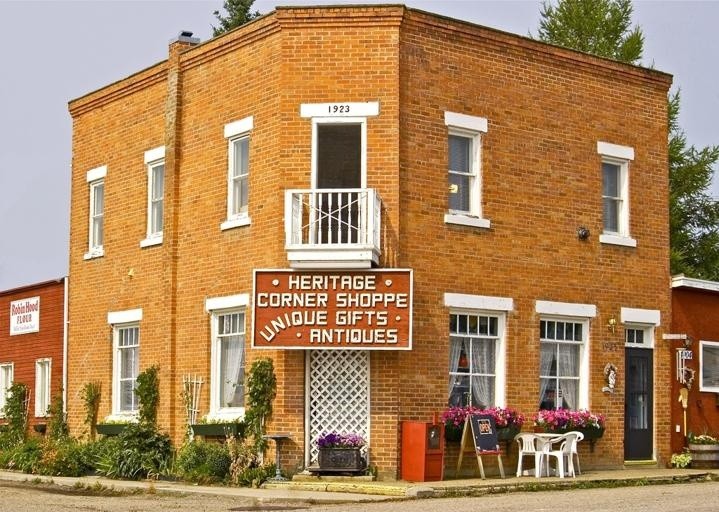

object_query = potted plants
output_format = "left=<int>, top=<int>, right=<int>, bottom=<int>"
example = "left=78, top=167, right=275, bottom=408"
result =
left=689, top=443, right=719, bottom=469
left=191, top=416, right=246, bottom=436
left=95, top=419, right=140, bottom=436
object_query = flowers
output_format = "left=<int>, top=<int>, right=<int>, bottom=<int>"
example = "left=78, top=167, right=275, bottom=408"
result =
left=671, top=452, right=692, bottom=469
left=442, top=406, right=605, bottom=432
left=318, top=433, right=366, bottom=448
left=687, top=433, right=718, bottom=445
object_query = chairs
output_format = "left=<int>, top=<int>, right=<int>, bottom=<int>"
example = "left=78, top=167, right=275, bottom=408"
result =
left=512, top=430, right=585, bottom=478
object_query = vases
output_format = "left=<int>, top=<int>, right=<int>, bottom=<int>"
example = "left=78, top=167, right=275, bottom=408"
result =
left=318, top=447, right=361, bottom=469
